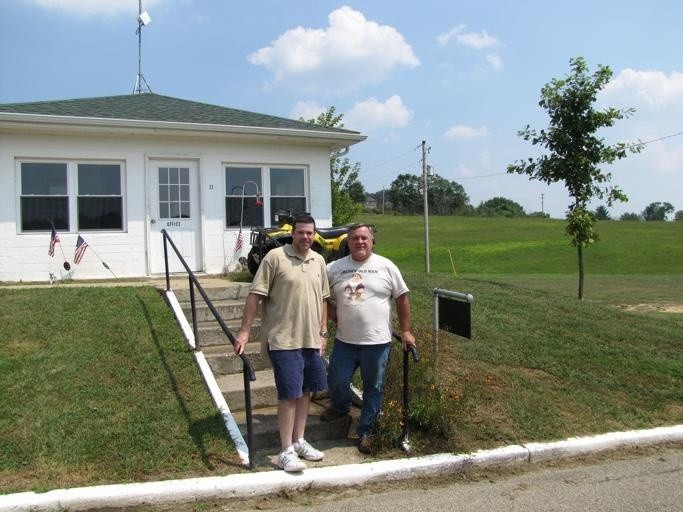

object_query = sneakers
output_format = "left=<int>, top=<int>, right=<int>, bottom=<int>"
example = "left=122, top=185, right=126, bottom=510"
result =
left=293, top=440, right=325, bottom=461
left=276, top=446, right=306, bottom=472
left=359, top=438, right=371, bottom=452
left=320, top=407, right=350, bottom=421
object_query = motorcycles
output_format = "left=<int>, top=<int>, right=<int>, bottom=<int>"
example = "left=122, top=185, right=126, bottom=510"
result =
left=247, top=209, right=377, bottom=277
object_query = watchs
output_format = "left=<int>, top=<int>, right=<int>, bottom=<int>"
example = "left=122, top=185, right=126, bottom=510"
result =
left=318, top=331, right=330, bottom=338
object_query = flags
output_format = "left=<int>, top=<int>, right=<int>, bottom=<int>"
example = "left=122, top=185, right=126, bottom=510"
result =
left=72, top=235, right=88, bottom=265
left=235, top=227, right=245, bottom=254
left=46, top=224, right=60, bottom=258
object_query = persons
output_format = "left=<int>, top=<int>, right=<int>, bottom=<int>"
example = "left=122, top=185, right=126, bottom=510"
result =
left=230, top=212, right=332, bottom=474
left=320, top=222, right=418, bottom=454
